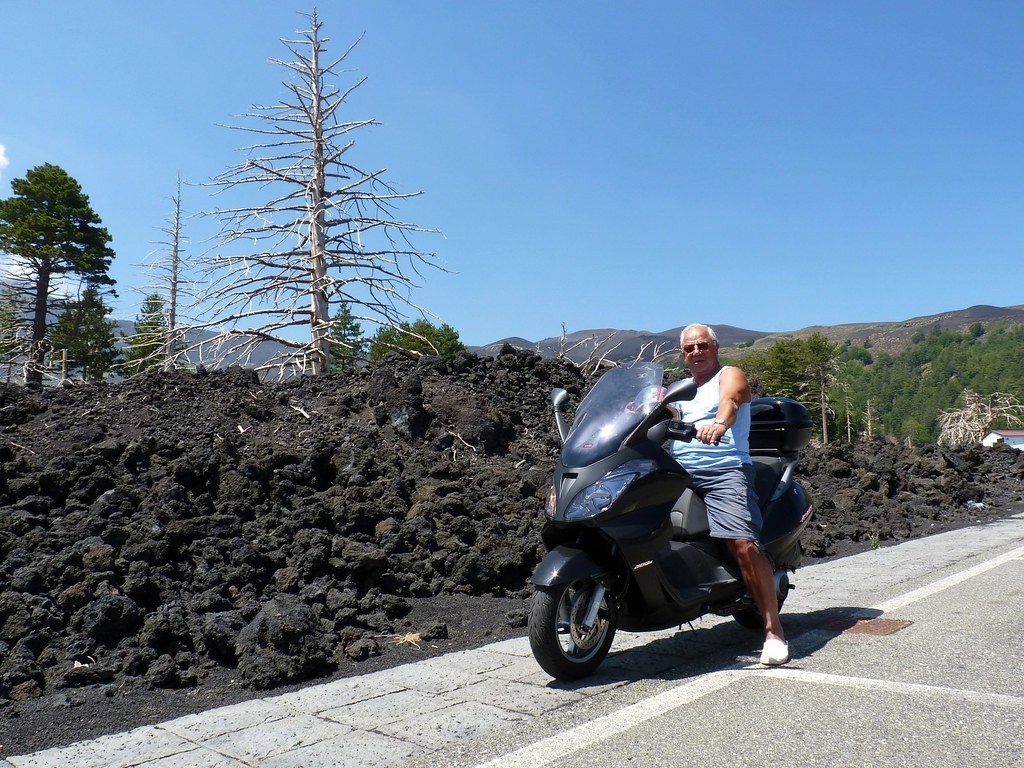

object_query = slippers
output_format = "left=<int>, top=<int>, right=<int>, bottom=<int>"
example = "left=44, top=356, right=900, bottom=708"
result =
left=759, top=640, right=789, bottom=664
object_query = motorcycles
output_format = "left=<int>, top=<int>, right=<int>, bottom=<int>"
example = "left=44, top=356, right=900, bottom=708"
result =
left=525, top=360, right=816, bottom=681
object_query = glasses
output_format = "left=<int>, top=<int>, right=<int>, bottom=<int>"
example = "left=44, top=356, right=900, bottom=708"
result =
left=684, top=342, right=712, bottom=353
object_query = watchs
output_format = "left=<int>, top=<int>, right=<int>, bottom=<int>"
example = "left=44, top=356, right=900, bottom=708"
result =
left=712, top=417, right=728, bottom=428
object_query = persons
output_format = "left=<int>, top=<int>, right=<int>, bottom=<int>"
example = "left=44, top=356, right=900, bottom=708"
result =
left=622, top=324, right=790, bottom=665
